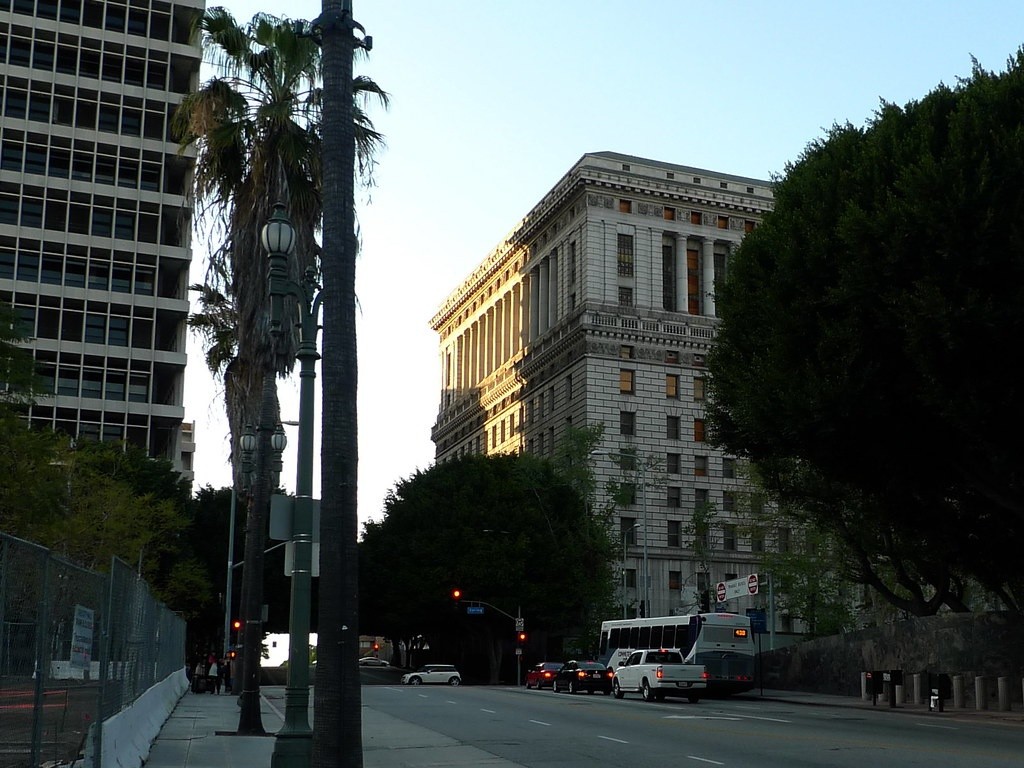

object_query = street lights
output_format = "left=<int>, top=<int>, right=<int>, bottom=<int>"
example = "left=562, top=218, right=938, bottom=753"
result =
left=258, top=205, right=326, bottom=768
left=591, top=450, right=649, bottom=618
left=220, top=420, right=301, bottom=695
left=231, top=421, right=287, bottom=697
left=622, top=523, right=644, bottom=618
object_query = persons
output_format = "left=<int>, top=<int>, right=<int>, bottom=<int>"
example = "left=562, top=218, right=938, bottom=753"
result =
left=207, top=649, right=237, bottom=694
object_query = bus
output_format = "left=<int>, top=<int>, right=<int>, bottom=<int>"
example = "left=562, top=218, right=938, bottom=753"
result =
left=598, top=612, right=756, bottom=699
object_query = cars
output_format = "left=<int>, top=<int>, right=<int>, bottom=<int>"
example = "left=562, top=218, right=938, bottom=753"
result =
left=400, top=664, right=462, bottom=688
left=525, top=662, right=564, bottom=690
left=553, top=660, right=612, bottom=695
left=359, top=656, right=390, bottom=668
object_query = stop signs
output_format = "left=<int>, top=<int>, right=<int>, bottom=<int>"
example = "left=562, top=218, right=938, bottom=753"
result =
left=717, top=583, right=726, bottom=601
left=748, top=575, right=758, bottom=594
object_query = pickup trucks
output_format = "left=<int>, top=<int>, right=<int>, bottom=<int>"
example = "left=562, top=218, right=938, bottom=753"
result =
left=611, top=647, right=708, bottom=703
